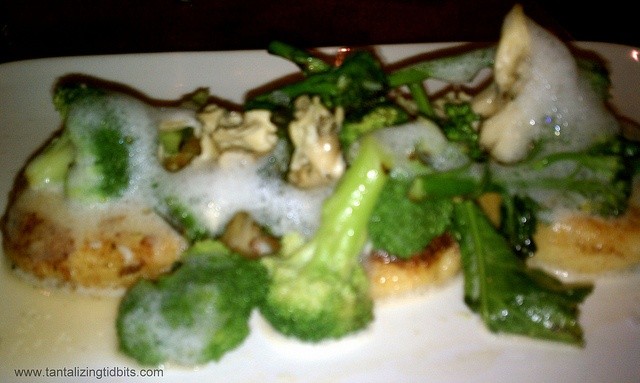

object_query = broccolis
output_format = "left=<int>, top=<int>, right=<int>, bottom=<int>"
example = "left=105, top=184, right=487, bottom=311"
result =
left=260, top=139, right=390, bottom=343
left=115, top=236, right=268, bottom=367
left=369, top=179, right=456, bottom=263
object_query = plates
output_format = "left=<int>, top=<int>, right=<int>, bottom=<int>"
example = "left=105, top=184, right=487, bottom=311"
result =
left=1, top=41, right=636, bottom=382
